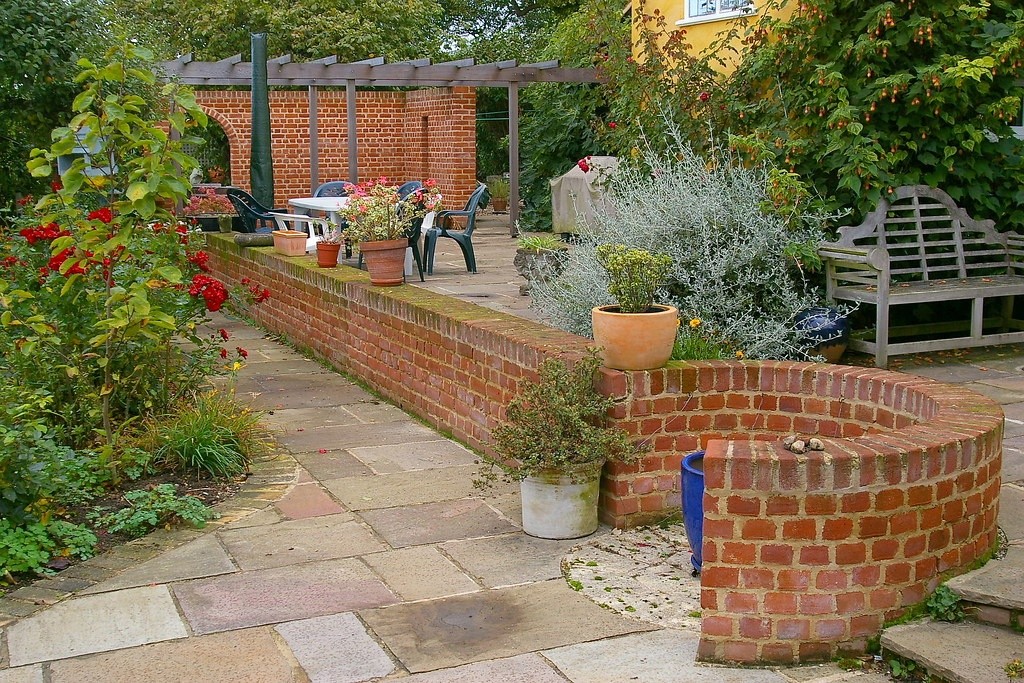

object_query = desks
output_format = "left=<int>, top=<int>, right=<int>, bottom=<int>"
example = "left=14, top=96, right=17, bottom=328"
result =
left=289, top=196, right=351, bottom=265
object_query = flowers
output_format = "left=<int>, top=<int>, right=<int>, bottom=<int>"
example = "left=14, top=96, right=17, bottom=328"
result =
left=180, top=186, right=235, bottom=215
left=332, top=174, right=443, bottom=241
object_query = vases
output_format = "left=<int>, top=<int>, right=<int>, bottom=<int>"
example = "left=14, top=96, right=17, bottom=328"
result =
left=360, top=238, right=408, bottom=287
left=272, top=230, right=308, bottom=257
left=184, top=215, right=240, bottom=231
left=790, top=308, right=849, bottom=363
left=680, top=452, right=704, bottom=577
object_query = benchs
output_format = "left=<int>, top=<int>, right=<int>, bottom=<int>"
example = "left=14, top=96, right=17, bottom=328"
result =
left=818, top=184, right=1024, bottom=369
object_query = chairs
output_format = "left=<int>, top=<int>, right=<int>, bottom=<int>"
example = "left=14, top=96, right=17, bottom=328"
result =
left=268, top=211, right=330, bottom=252
left=227, top=187, right=290, bottom=233
left=305, top=181, right=357, bottom=258
left=305, top=181, right=486, bottom=276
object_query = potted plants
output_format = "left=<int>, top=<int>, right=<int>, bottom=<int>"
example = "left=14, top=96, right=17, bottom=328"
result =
left=489, top=179, right=510, bottom=211
left=514, top=234, right=571, bottom=296
left=471, top=344, right=654, bottom=540
left=590, top=242, right=677, bottom=371
left=312, top=220, right=341, bottom=268
